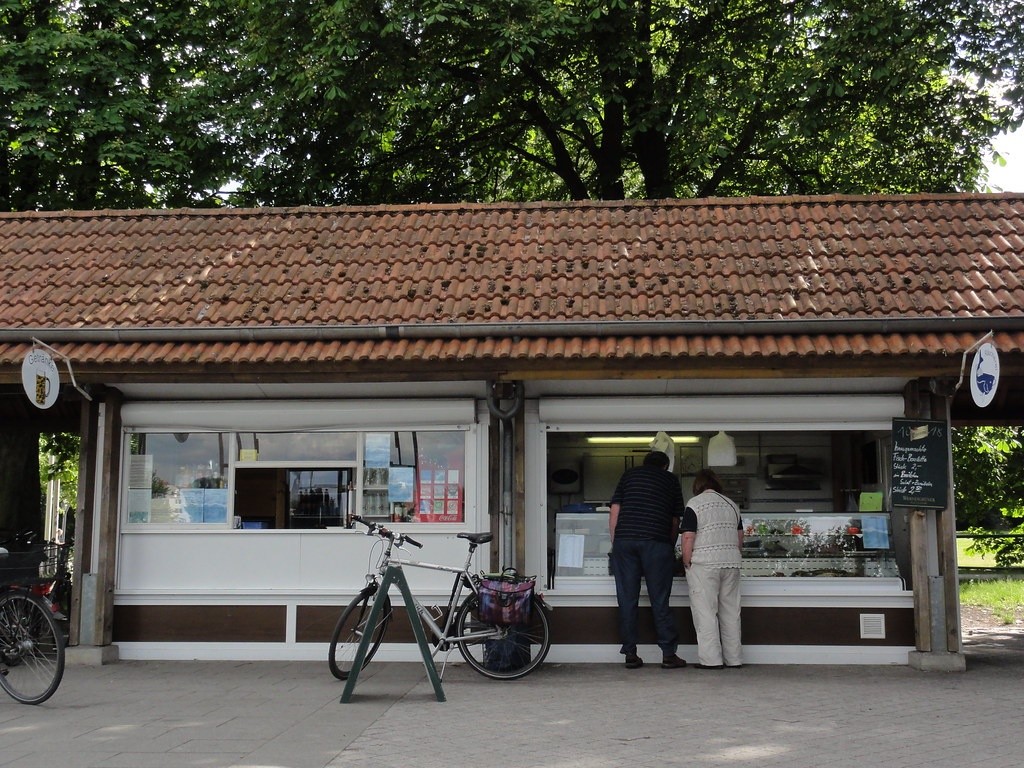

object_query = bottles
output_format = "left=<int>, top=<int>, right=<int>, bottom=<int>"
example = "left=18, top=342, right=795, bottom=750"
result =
left=394, top=503, right=408, bottom=522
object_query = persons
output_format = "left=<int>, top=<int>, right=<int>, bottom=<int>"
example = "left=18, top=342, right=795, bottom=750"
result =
left=610, top=452, right=744, bottom=668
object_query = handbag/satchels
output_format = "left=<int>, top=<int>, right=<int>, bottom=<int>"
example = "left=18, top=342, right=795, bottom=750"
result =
left=478, top=568, right=535, bottom=625
left=482, top=624, right=531, bottom=671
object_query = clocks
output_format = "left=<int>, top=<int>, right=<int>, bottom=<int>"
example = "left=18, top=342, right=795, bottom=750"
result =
left=683, top=454, right=702, bottom=473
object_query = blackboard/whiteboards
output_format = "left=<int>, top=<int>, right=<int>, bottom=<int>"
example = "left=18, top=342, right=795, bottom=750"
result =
left=890, top=416, right=949, bottom=509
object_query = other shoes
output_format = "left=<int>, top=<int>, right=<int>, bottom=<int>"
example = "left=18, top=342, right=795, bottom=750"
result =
left=728, top=665, right=741, bottom=668
left=695, top=664, right=723, bottom=669
left=626, top=656, right=643, bottom=667
left=662, top=654, right=687, bottom=667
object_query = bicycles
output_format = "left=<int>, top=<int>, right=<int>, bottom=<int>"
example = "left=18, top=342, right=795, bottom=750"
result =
left=326, top=513, right=555, bottom=682
left=0, top=544, right=67, bottom=705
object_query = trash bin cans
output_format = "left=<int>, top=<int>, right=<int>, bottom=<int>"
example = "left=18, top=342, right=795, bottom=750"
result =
left=477, top=591, right=553, bottom=674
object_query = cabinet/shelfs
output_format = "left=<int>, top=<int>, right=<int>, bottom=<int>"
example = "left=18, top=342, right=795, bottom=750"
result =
left=285, top=468, right=352, bottom=529
left=549, top=512, right=907, bottom=589
left=354, top=487, right=390, bottom=518
